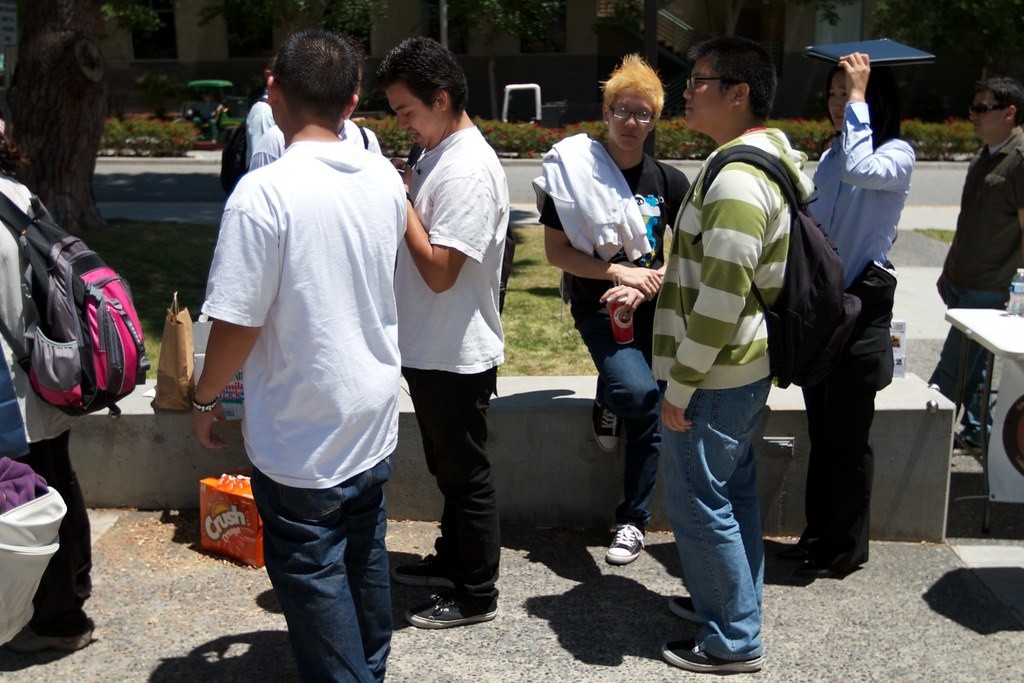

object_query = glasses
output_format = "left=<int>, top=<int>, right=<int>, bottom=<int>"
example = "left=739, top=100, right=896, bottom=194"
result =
left=609, top=104, right=655, bottom=123
left=687, top=75, right=738, bottom=89
left=969, top=100, right=1009, bottom=115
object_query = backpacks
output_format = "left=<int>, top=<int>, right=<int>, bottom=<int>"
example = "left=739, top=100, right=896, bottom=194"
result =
left=0, top=186, right=149, bottom=416
left=220, top=97, right=269, bottom=191
left=700, top=141, right=864, bottom=388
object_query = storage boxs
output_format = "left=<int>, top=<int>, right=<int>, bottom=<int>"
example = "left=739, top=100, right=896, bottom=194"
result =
left=200, top=478, right=264, bottom=568
left=193, top=322, right=245, bottom=420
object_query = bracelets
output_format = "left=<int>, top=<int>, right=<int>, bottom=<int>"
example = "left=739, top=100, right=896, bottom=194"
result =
left=406, top=192, right=413, bottom=206
left=193, top=394, right=220, bottom=412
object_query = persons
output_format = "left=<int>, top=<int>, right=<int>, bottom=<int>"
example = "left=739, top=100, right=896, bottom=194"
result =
left=193, top=27, right=407, bottom=683
left=373, top=35, right=511, bottom=630
left=928, top=75, right=1024, bottom=449
left=0, top=115, right=94, bottom=672
left=774, top=50, right=915, bottom=578
left=531, top=52, right=691, bottom=565
left=651, top=34, right=812, bottom=673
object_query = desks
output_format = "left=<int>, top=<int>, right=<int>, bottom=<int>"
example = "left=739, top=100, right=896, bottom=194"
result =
left=945, top=307, right=1024, bottom=533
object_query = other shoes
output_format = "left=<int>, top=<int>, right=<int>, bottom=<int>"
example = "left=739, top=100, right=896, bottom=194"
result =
left=774, top=545, right=811, bottom=562
left=961, top=423, right=982, bottom=447
left=792, top=561, right=848, bottom=576
left=11, top=618, right=96, bottom=652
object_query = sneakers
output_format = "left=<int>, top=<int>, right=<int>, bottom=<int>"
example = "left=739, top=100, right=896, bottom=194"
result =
left=391, top=553, right=452, bottom=587
left=404, top=592, right=497, bottom=628
left=669, top=594, right=708, bottom=625
left=591, top=394, right=622, bottom=451
left=606, top=523, right=646, bottom=564
left=662, top=637, right=762, bottom=672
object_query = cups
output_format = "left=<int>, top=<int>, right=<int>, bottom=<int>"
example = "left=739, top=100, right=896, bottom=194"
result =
left=606, top=295, right=634, bottom=344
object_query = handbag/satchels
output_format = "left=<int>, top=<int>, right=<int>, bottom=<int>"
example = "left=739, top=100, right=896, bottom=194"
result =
left=155, top=290, right=193, bottom=414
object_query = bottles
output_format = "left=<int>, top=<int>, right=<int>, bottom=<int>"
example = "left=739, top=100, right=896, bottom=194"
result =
left=1006, top=268, right=1024, bottom=315
